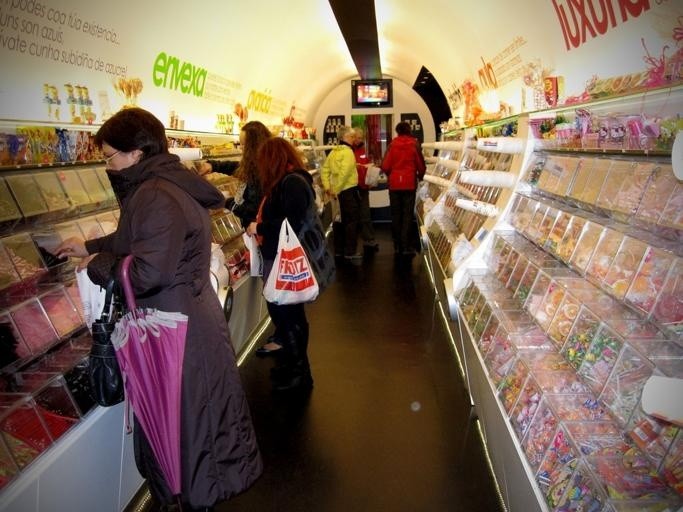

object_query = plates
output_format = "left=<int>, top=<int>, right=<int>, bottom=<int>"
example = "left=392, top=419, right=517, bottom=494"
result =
left=416, top=127, right=464, bottom=296
left=418, top=113, right=528, bottom=352
left=1, top=149, right=334, bottom=512
left=431, top=79, right=683, bottom=512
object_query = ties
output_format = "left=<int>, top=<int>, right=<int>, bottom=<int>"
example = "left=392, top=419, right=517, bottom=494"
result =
left=351, top=79, right=393, bottom=108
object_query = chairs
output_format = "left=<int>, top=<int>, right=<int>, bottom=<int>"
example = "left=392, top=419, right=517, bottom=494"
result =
left=254, top=344, right=285, bottom=357
left=275, top=374, right=310, bottom=393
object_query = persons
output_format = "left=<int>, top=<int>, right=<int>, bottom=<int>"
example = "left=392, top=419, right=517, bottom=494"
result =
left=353, top=127, right=378, bottom=247
left=321, top=127, right=363, bottom=259
left=52, top=109, right=264, bottom=512
left=195, top=122, right=272, bottom=226
left=247, top=136, right=337, bottom=393
left=381, top=122, right=427, bottom=256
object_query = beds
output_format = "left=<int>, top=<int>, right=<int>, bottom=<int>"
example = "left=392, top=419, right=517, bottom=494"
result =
left=111, top=252, right=188, bottom=512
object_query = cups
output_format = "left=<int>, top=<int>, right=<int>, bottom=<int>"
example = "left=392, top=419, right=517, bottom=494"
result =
left=103, top=151, right=122, bottom=165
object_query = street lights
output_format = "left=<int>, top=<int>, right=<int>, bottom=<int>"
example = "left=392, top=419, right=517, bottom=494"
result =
left=88, top=273, right=129, bottom=407
left=240, top=232, right=263, bottom=277
left=323, top=192, right=344, bottom=223
left=282, top=172, right=337, bottom=305
left=362, top=162, right=380, bottom=188
